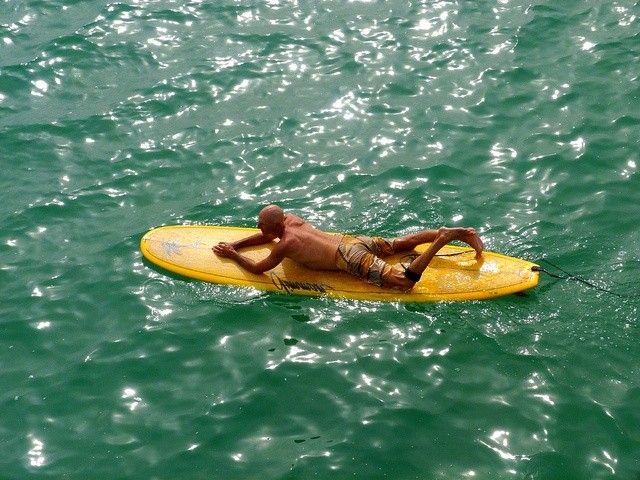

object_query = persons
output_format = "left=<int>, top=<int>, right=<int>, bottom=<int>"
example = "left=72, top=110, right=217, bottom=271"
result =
left=212, top=204, right=484, bottom=291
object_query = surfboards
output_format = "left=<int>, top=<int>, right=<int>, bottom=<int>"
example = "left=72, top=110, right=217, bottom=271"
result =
left=141, top=225, right=542, bottom=301
